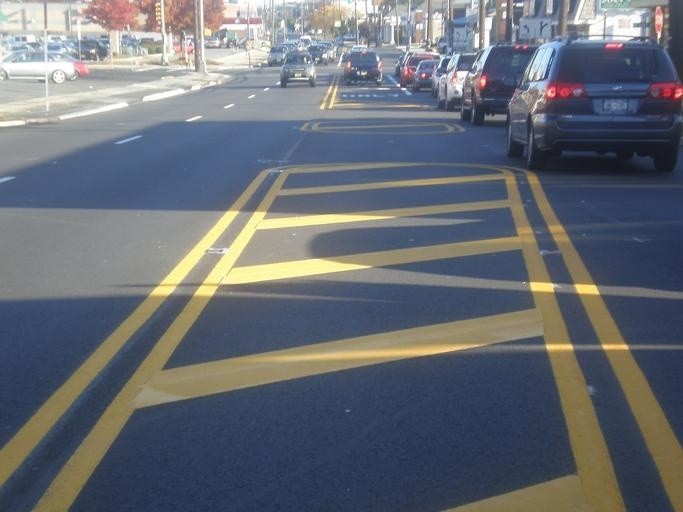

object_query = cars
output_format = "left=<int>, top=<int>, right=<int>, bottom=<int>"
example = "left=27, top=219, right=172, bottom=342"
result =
left=0, top=29, right=246, bottom=83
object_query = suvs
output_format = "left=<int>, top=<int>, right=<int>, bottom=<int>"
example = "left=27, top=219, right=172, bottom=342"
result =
left=266, top=30, right=481, bottom=111
left=502, top=34, right=682, bottom=178
left=458, top=39, right=548, bottom=127
